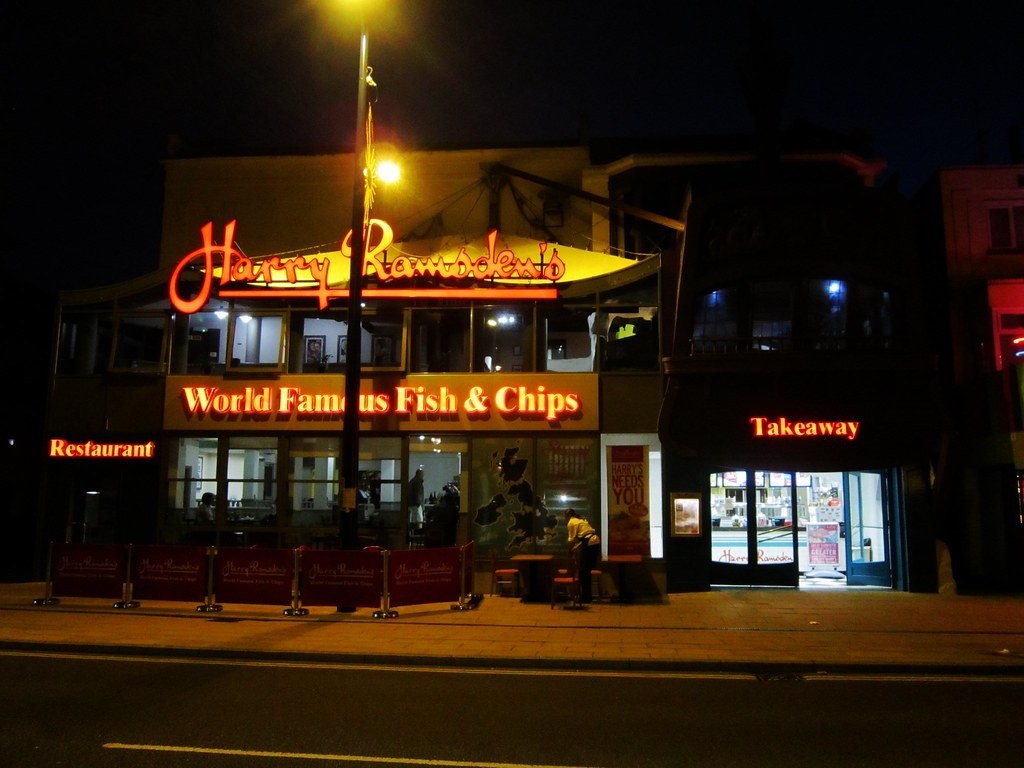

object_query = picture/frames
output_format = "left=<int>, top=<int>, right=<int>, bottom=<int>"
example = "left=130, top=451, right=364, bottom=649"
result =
left=304, top=335, right=326, bottom=364
left=337, top=335, right=347, bottom=363
left=371, top=335, right=395, bottom=361
left=196, top=457, right=203, bottom=490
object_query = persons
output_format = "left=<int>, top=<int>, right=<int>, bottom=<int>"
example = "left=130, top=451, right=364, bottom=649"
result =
left=409, top=468, right=425, bottom=522
left=197, top=492, right=213, bottom=521
left=308, top=342, right=320, bottom=363
left=369, top=471, right=381, bottom=510
left=565, top=509, right=597, bottom=604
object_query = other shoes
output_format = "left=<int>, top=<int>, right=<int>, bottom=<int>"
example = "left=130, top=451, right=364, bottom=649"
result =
left=576, top=596, right=593, bottom=604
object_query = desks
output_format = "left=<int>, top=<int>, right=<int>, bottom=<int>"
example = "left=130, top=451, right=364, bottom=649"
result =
left=510, top=553, right=555, bottom=604
left=598, top=555, right=642, bottom=604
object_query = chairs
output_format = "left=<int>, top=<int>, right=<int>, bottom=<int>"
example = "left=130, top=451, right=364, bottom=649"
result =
left=488, top=548, right=520, bottom=598
left=551, top=551, right=603, bottom=610
